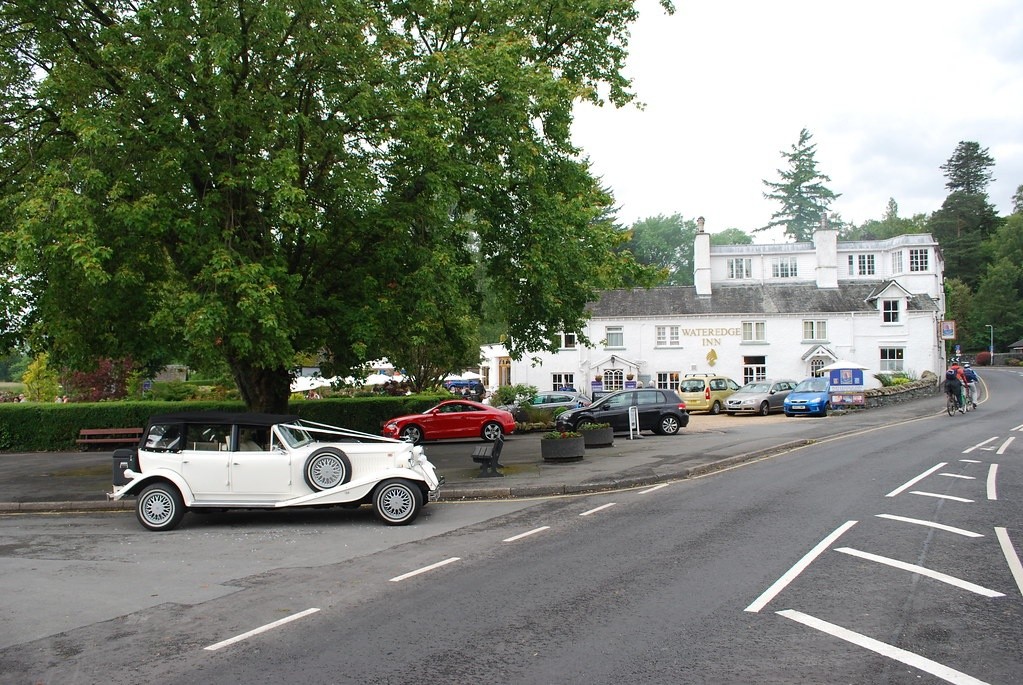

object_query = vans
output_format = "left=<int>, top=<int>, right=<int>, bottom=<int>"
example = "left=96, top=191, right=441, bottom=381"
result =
left=446, top=381, right=483, bottom=401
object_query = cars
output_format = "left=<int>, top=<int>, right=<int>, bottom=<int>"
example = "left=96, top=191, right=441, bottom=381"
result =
left=678, top=373, right=741, bottom=415
left=104, top=408, right=446, bottom=533
left=783, top=376, right=831, bottom=417
left=495, top=392, right=587, bottom=424
left=723, top=378, right=798, bottom=416
left=554, top=388, right=689, bottom=436
left=381, top=400, right=516, bottom=446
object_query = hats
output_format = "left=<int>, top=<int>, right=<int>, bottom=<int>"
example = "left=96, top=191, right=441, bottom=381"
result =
left=964, top=364, right=970, bottom=368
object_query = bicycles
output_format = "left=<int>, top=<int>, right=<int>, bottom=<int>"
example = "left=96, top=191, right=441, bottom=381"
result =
left=947, top=379, right=979, bottom=417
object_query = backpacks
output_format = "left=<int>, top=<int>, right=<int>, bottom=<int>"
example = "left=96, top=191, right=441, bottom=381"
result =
left=963, top=370, right=972, bottom=381
left=946, top=369, right=957, bottom=380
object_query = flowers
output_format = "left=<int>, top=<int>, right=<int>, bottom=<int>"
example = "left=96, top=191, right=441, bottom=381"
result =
left=543, top=431, right=577, bottom=439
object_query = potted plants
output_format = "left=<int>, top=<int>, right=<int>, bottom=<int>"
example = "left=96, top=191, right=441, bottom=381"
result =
left=577, top=422, right=614, bottom=448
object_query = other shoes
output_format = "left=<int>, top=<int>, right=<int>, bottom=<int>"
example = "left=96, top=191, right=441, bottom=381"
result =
left=959, top=408, right=963, bottom=412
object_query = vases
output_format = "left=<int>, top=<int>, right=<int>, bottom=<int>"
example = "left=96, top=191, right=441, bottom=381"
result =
left=541, top=436, right=585, bottom=462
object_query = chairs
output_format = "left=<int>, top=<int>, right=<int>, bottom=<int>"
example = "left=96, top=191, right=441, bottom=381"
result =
left=225, top=432, right=263, bottom=450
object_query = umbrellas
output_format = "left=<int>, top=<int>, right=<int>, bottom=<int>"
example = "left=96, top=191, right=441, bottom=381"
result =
left=461, top=372, right=486, bottom=387
left=431, top=372, right=462, bottom=388
left=292, top=373, right=414, bottom=392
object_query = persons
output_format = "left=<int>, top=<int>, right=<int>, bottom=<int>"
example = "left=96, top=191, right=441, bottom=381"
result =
left=944, top=362, right=968, bottom=412
left=475, top=380, right=484, bottom=394
left=559, top=385, right=566, bottom=391
left=0, top=393, right=27, bottom=402
left=54, top=395, right=69, bottom=403
left=565, top=383, right=574, bottom=391
left=961, top=364, right=980, bottom=413
left=464, top=388, right=471, bottom=395
left=456, top=388, right=462, bottom=395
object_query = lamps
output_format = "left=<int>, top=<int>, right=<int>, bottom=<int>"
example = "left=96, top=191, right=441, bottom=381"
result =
left=610, top=355, right=615, bottom=363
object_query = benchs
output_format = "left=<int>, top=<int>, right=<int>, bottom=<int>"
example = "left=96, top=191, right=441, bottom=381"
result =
left=75, top=428, right=143, bottom=452
left=471, top=438, right=505, bottom=477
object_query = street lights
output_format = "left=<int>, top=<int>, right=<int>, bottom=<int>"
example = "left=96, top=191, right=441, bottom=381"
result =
left=985, top=324, right=993, bottom=367
